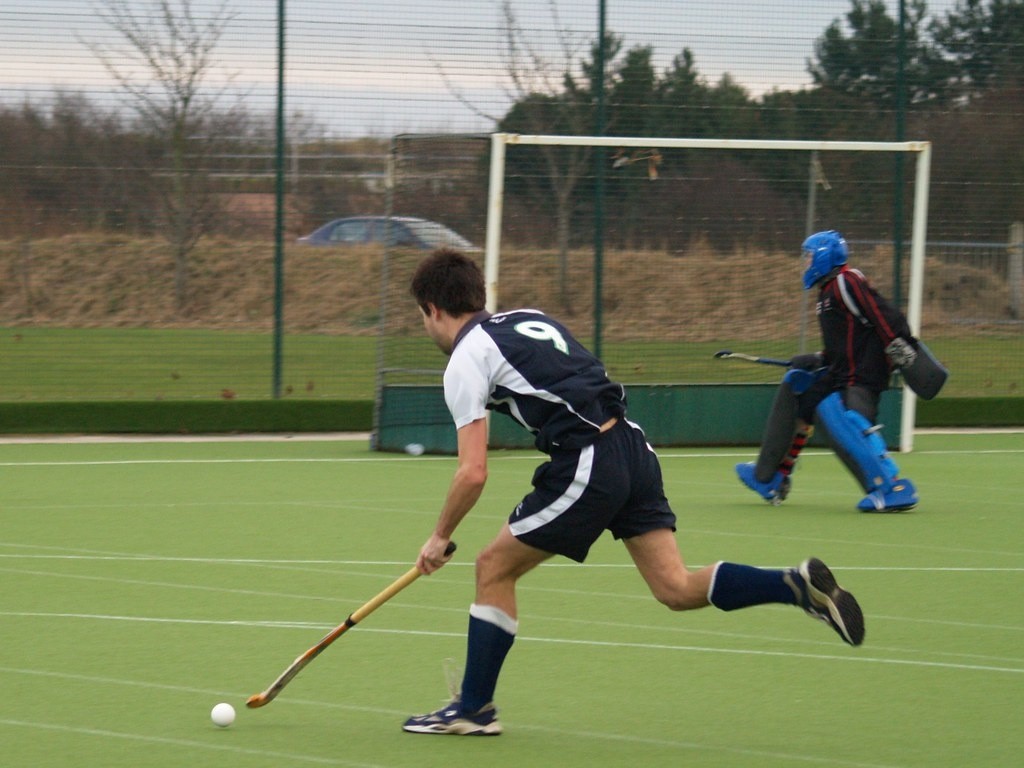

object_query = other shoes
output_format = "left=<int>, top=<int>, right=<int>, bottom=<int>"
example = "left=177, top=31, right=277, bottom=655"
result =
left=856, top=478, right=919, bottom=511
left=736, top=463, right=791, bottom=505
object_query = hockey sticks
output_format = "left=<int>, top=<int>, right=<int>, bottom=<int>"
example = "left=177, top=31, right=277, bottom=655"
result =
left=245, top=541, right=457, bottom=709
left=712, top=350, right=791, bottom=366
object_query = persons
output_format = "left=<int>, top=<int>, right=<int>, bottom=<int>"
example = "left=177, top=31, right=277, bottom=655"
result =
left=734, top=229, right=922, bottom=511
left=402, top=245, right=865, bottom=735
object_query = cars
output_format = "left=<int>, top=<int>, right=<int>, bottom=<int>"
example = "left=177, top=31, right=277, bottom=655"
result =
left=294, top=215, right=484, bottom=253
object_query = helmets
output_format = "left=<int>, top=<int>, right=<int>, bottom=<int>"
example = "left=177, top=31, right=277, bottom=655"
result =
left=798, top=230, right=848, bottom=289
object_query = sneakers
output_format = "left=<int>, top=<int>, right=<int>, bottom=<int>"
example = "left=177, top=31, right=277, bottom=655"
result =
left=783, top=556, right=865, bottom=647
left=402, top=699, right=502, bottom=736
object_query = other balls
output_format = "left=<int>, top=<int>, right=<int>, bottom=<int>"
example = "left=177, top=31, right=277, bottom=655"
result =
left=211, top=702, right=235, bottom=728
